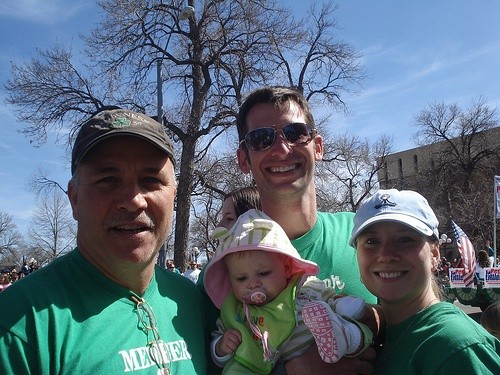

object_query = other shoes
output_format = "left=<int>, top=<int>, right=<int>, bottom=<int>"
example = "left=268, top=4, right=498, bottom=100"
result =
left=301, top=300, right=347, bottom=363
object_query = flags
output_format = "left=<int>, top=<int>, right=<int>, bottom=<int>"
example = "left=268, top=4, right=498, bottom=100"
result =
left=451, top=220, right=476, bottom=288
left=495, top=175, right=500, bottom=219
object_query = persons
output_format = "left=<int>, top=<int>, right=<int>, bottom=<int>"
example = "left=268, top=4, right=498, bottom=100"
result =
left=204, top=209, right=381, bottom=375
left=0, top=105, right=214, bottom=375
left=485, top=240, right=495, bottom=257
left=349, top=189, right=500, bottom=375
left=475, top=250, right=494, bottom=278
left=197, top=186, right=264, bottom=291
left=2, top=257, right=497, bottom=291
left=198, top=87, right=381, bottom=374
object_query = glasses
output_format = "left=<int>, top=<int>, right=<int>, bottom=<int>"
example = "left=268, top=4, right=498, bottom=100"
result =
left=131, top=295, right=172, bottom=375
left=238, top=123, right=318, bottom=152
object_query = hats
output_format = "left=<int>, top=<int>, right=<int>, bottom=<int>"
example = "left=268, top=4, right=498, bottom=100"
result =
left=349, top=189, right=440, bottom=248
left=71, top=107, right=174, bottom=174
